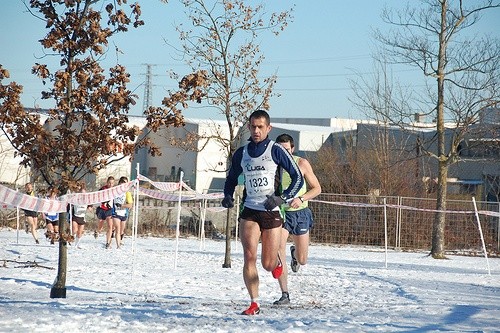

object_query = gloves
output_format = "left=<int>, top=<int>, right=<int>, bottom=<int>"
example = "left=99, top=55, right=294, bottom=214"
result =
left=115, top=203, right=121, bottom=207
left=221, top=195, right=234, bottom=208
left=264, top=195, right=285, bottom=211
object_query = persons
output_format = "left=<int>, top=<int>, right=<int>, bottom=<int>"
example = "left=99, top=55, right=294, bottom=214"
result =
left=272, top=133, right=321, bottom=308
left=222, top=109, right=305, bottom=316
left=21, top=176, right=134, bottom=250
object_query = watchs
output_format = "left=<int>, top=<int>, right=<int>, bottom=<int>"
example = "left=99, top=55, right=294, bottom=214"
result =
left=280, top=194, right=287, bottom=204
left=298, top=196, right=304, bottom=203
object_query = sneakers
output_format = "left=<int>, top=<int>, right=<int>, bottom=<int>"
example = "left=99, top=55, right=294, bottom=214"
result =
left=273, top=292, right=290, bottom=305
left=116, top=245, right=122, bottom=249
left=120, top=240, right=125, bottom=246
left=94, top=230, right=98, bottom=238
left=272, top=251, right=283, bottom=279
left=73, top=245, right=80, bottom=250
left=241, top=302, right=260, bottom=315
left=290, top=246, right=300, bottom=272
left=106, top=243, right=111, bottom=249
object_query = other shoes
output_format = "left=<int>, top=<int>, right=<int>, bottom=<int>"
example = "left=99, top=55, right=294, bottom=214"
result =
left=35, top=240, right=39, bottom=244
left=47, top=238, right=60, bottom=244
left=26, top=228, right=29, bottom=234
